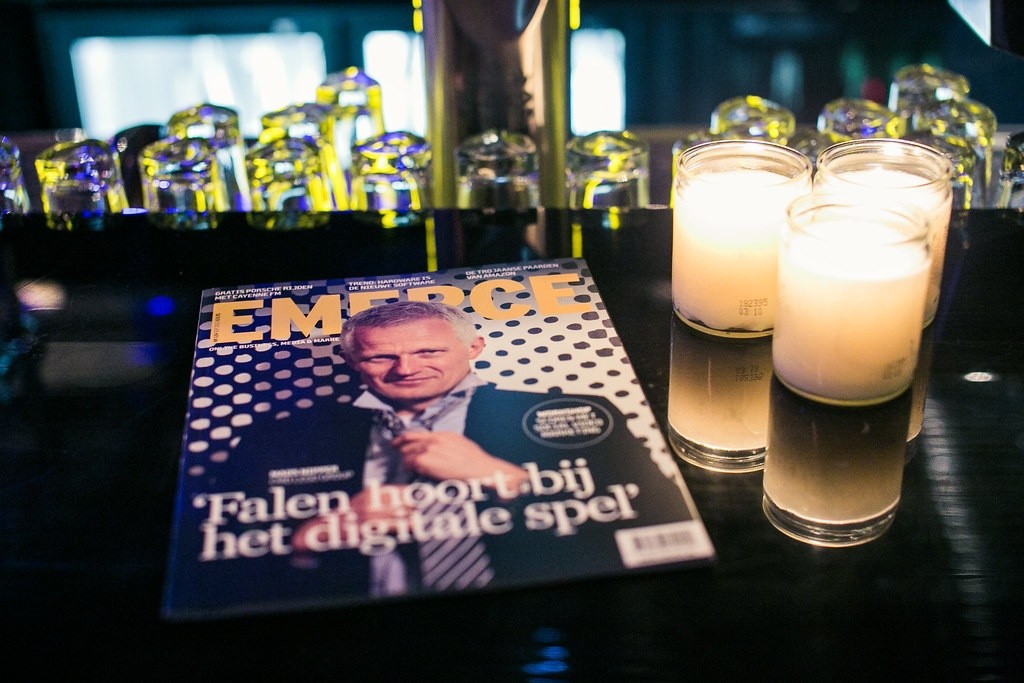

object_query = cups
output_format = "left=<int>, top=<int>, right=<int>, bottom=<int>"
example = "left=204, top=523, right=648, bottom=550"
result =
left=811, top=137, right=954, bottom=443
left=763, top=191, right=932, bottom=547
left=667, top=140, right=812, bottom=475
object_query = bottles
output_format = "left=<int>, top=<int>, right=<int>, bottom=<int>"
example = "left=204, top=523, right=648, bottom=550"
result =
left=0, top=63, right=1024, bottom=212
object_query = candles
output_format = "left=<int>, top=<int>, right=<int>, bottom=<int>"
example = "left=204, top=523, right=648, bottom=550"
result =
left=814, top=138, right=954, bottom=330
left=771, top=186, right=929, bottom=405
left=762, top=377, right=905, bottom=549
left=665, top=320, right=772, bottom=472
left=669, top=138, right=812, bottom=339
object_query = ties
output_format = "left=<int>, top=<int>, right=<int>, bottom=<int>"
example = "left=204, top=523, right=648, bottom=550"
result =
left=371, top=393, right=498, bottom=594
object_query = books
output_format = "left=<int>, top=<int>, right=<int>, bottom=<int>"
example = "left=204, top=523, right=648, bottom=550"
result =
left=167, top=254, right=715, bottom=630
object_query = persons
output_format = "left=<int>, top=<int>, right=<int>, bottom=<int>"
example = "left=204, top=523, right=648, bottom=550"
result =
left=181, top=299, right=691, bottom=607
